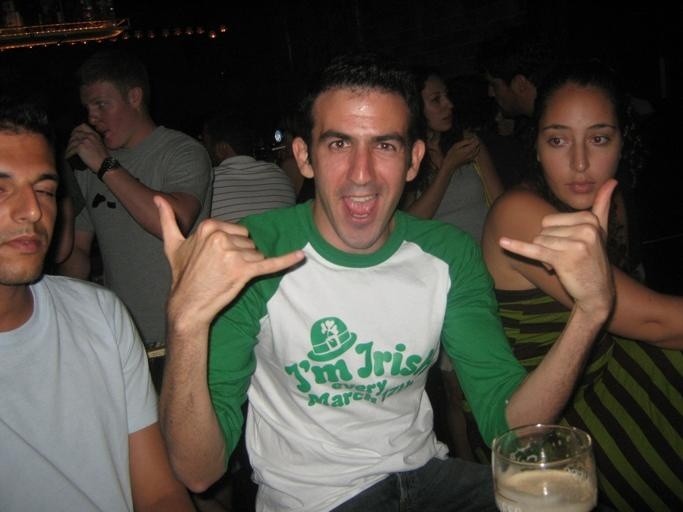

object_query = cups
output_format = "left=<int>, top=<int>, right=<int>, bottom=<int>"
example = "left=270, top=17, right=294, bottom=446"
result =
left=67, top=117, right=106, bottom=172
left=491, top=421, right=600, bottom=512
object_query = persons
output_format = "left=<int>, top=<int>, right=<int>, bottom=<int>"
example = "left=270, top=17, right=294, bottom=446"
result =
left=58, top=52, right=213, bottom=396
left=402, top=62, right=504, bottom=458
left=201, top=117, right=312, bottom=224
left=152, top=57, right=617, bottom=512
left=43, top=137, right=87, bottom=275
left=482, top=61, right=683, bottom=510
left=2, top=94, right=196, bottom=512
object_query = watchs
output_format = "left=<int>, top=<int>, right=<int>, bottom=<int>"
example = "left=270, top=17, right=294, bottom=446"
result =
left=97, top=156, right=120, bottom=183
